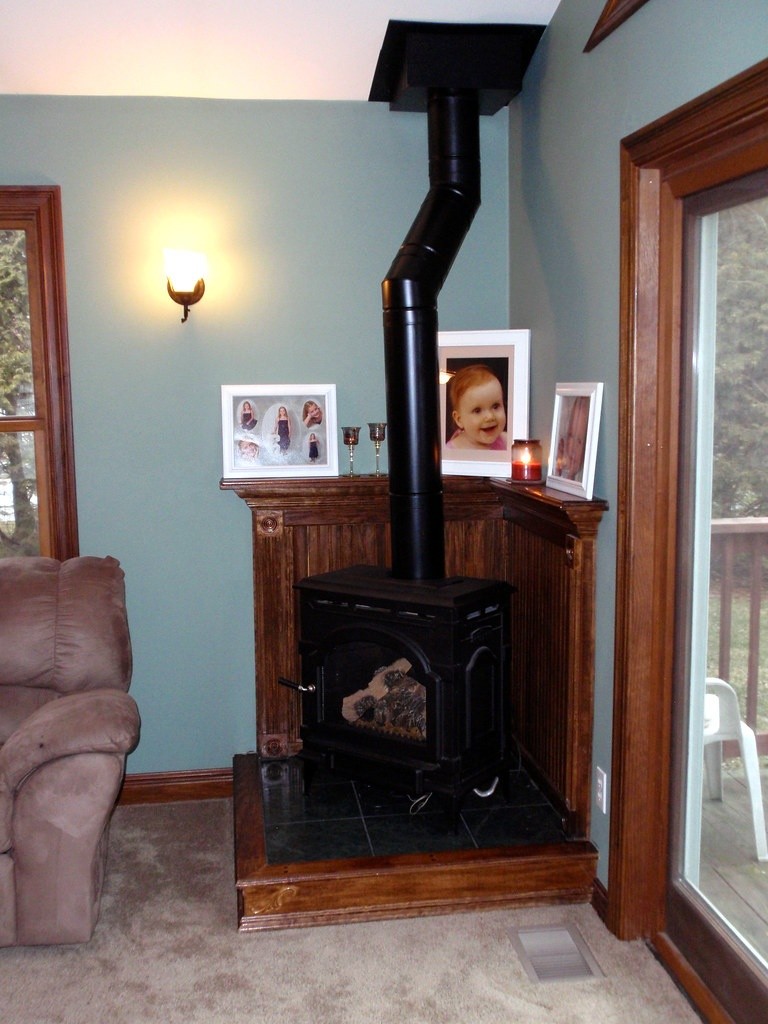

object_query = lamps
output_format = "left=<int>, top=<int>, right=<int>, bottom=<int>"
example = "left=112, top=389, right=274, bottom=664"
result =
left=161, top=248, right=208, bottom=322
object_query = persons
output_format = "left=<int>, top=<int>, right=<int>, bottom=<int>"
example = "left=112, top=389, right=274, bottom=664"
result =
left=241, top=401, right=258, bottom=430
left=555, top=396, right=590, bottom=482
left=272, top=406, right=292, bottom=455
left=303, top=401, right=322, bottom=428
left=447, top=365, right=508, bottom=450
left=309, top=433, right=319, bottom=462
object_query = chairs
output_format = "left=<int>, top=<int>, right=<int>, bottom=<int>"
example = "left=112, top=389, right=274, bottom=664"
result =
left=703, top=677, right=768, bottom=861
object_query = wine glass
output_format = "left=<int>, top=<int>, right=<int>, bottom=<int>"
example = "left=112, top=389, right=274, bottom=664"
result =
left=341, top=426, right=361, bottom=477
left=367, top=423, right=388, bottom=477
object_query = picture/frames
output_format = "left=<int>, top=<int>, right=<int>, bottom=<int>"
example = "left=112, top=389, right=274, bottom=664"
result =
left=220, top=383, right=339, bottom=477
left=545, top=381, right=604, bottom=499
left=432, top=329, right=530, bottom=478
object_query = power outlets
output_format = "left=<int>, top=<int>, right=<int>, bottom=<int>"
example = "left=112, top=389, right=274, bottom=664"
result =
left=595, top=767, right=607, bottom=815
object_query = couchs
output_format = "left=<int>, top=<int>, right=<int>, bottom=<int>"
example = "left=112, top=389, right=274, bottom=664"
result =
left=0, top=556, right=142, bottom=946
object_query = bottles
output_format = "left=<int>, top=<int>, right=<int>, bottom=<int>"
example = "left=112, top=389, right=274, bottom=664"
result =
left=511, top=440, right=543, bottom=481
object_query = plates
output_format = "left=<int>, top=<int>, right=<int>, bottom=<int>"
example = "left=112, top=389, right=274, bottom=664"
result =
left=506, top=478, right=546, bottom=485
